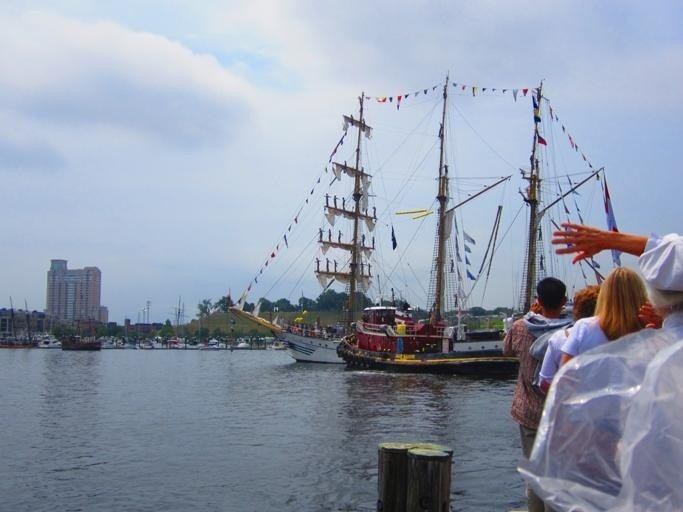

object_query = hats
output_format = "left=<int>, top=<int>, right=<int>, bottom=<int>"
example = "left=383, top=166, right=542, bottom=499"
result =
left=637, top=233, right=683, bottom=292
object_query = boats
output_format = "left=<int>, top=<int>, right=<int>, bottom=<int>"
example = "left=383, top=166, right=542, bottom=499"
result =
left=0, top=335, right=101, bottom=350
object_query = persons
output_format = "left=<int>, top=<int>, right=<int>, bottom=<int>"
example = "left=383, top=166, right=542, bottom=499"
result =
left=501, top=278, right=577, bottom=512
left=553, top=220, right=665, bottom=331
left=333, top=259, right=338, bottom=272
left=338, top=230, right=344, bottom=243
left=283, top=318, right=343, bottom=343
left=317, top=228, right=326, bottom=243
left=560, top=265, right=665, bottom=367
left=333, top=195, right=338, bottom=209
left=329, top=228, right=331, bottom=242
left=518, top=232, right=682, bottom=512
left=315, top=257, right=322, bottom=271
left=322, top=193, right=331, bottom=206
left=536, top=285, right=600, bottom=394
left=326, top=257, right=331, bottom=272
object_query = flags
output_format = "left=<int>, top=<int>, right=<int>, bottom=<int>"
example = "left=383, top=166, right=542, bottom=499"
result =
left=599, top=171, right=622, bottom=270
left=391, top=225, right=397, bottom=250
